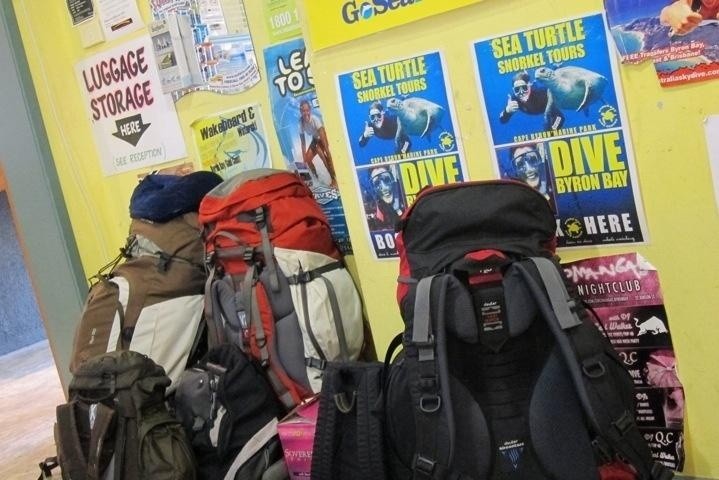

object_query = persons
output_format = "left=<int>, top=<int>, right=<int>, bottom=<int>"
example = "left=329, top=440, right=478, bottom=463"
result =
left=499, top=73, right=565, bottom=131
left=660, top=0, right=719, bottom=34
left=297, top=100, right=338, bottom=191
left=508, top=145, right=558, bottom=217
left=368, top=166, right=407, bottom=229
left=358, top=101, right=412, bottom=154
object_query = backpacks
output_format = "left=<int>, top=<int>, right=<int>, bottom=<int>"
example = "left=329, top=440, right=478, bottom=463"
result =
left=166, top=344, right=290, bottom=480
left=379, top=175, right=677, bottom=480
left=50, top=346, right=197, bottom=480
left=74, top=211, right=226, bottom=406
left=196, top=165, right=370, bottom=415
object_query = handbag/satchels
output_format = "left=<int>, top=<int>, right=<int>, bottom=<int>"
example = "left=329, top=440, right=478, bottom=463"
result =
left=125, top=169, right=226, bottom=223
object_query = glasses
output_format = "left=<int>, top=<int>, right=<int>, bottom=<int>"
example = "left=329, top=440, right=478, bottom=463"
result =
left=370, top=170, right=392, bottom=188
left=511, top=83, right=530, bottom=95
left=509, top=149, right=543, bottom=174
left=369, top=113, right=383, bottom=121
